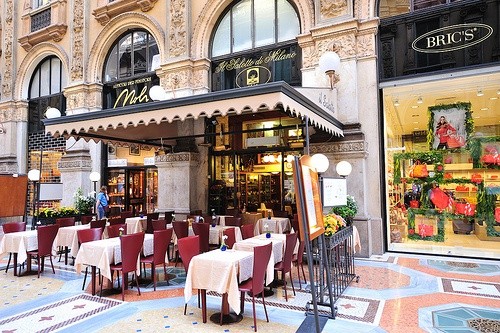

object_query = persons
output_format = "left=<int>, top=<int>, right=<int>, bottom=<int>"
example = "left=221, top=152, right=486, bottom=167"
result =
left=435, top=116, right=457, bottom=150
left=96, top=186, right=109, bottom=220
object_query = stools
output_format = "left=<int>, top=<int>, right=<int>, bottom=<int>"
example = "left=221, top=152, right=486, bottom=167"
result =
left=453, top=219, right=474, bottom=235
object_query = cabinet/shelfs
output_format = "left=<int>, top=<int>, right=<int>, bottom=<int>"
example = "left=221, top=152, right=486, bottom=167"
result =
left=388, top=138, right=500, bottom=244
left=240, top=172, right=281, bottom=214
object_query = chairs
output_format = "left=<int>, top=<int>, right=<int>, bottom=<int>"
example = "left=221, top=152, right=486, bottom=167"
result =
left=2, top=209, right=307, bottom=332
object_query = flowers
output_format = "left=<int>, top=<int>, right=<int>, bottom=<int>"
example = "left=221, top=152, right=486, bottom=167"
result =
left=312, top=213, right=347, bottom=237
left=34, top=186, right=96, bottom=225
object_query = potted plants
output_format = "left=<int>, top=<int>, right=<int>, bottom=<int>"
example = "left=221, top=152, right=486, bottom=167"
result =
left=329, top=195, right=357, bottom=226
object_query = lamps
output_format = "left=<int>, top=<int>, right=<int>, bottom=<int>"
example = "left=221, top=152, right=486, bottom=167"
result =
left=319, top=51, right=340, bottom=89
left=45, top=107, right=61, bottom=119
left=149, top=85, right=165, bottom=102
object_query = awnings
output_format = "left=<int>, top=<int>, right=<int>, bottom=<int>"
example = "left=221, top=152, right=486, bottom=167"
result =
left=39, top=80, right=344, bottom=161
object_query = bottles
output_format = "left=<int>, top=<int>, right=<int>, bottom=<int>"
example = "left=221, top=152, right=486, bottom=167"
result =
left=119, top=227, right=124, bottom=236
left=119, top=197, right=124, bottom=205
left=111, top=176, right=125, bottom=184
left=268, top=210, right=271, bottom=220
left=114, top=187, right=117, bottom=194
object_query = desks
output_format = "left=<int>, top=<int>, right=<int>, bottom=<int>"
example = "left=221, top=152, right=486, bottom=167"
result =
left=254, top=217, right=295, bottom=237
left=167, top=223, right=242, bottom=263
left=232, top=233, right=300, bottom=297
left=0, top=230, right=38, bottom=276
left=74, top=234, right=169, bottom=296
left=184, top=249, right=254, bottom=325
left=125, top=216, right=165, bottom=234
left=52, top=222, right=110, bottom=265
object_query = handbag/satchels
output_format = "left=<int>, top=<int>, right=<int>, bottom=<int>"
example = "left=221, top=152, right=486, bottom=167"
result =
left=409, top=160, right=429, bottom=177
left=446, top=134, right=460, bottom=148
left=453, top=198, right=475, bottom=235
left=482, top=147, right=500, bottom=165
left=418, top=224, right=433, bottom=239
left=103, top=206, right=112, bottom=213
